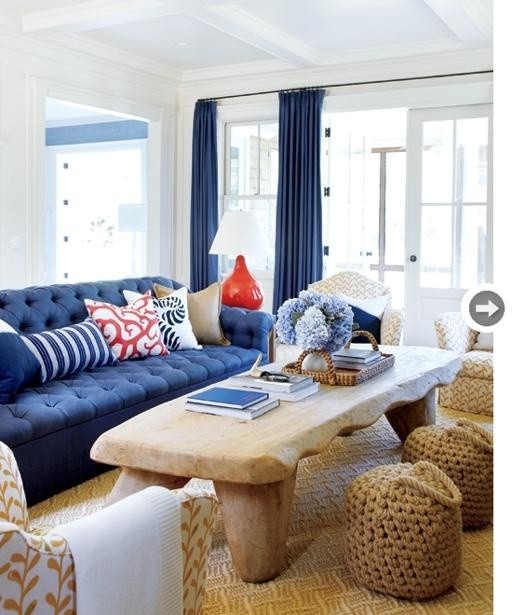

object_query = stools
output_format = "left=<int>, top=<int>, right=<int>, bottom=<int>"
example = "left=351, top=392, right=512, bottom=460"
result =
left=338, top=463, right=464, bottom=601
left=399, top=419, right=493, bottom=531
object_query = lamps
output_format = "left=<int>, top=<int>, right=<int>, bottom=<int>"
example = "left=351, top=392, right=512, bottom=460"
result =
left=204, top=209, right=275, bottom=310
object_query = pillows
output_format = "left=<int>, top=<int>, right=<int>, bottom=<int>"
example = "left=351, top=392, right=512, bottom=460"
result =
left=336, top=288, right=388, bottom=344
left=472, top=332, right=493, bottom=350
left=0, top=283, right=227, bottom=401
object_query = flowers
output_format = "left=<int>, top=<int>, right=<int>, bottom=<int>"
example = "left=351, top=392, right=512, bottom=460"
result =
left=275, top=286, right=352, bottom=350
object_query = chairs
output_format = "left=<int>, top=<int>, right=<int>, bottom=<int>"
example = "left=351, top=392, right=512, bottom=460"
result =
left=433, top=306, right=493, bottom=417
left=0, top=378, right=219, bottom=615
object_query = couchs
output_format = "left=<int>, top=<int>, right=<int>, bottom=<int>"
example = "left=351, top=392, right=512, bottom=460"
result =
left=0, top=274, right=277, bottom=509
left=293, top=268, right=399, bottom=350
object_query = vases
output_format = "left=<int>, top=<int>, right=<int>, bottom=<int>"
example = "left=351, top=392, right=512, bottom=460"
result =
left=290, top=345, right=338, bottom=374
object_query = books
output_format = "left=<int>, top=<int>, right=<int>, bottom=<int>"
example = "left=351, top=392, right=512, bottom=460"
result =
left=185, top=387, right=279, bottom=422
left=228, top=369, right=320, bottom=401
left=332, top=347, right=383, bottom=371
